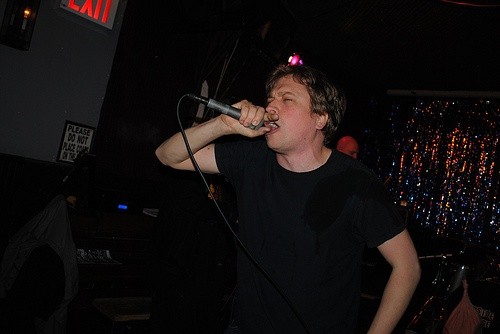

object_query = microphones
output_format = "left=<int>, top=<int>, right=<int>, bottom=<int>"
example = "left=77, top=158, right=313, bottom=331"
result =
left=192, top=93, right=265, bottom=132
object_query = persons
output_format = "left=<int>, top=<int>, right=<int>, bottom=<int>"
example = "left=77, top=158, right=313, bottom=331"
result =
left=153, top=63, right=421, bottom=334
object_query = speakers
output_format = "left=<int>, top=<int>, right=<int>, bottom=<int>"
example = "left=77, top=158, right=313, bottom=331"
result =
left=0, top=0, right=42, bottom=51
